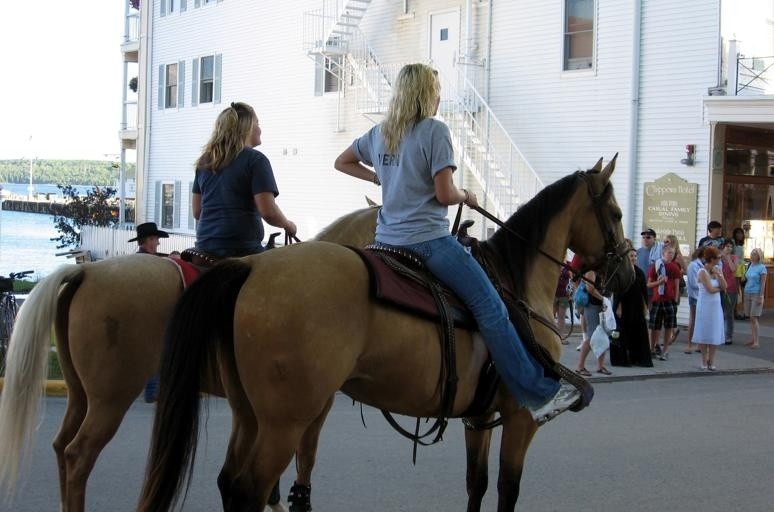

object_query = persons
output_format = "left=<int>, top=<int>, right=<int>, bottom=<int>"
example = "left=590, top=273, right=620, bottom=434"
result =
left=190, top=101, right=297, bottom=260
left=126, top=221, right=171, bottom=404
left=551, top=220, right=767, bottom=377
left=333, top=61, right=582, bottom=426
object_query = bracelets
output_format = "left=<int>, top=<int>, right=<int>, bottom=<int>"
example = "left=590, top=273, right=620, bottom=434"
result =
left=459, top=189, right=470, bottom=204
left=373, top=171, right=380, bottom=186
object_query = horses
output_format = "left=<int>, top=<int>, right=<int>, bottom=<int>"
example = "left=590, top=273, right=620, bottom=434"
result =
left=134, top=151, right=637, bottom=512
left=0, top=194, right=383, bottom=512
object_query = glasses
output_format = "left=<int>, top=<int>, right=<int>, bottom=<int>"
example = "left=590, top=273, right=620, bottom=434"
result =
left=643, top=237, right=650, bottom=239
left=664, top=241, right=669, bottom=243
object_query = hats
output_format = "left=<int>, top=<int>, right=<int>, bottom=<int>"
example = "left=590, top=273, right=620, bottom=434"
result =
left=128, top=223, right=169, bottom=242
left=641, top=229, right=656, bottom=238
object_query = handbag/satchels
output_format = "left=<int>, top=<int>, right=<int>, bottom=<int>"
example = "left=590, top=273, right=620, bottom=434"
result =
left=573, top=281, right=589, bottom=307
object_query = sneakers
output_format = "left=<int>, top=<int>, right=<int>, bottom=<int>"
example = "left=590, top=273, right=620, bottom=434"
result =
left=562, top=327, right=759, bottom=375
left=528, top=378, right=582, bottom=427
